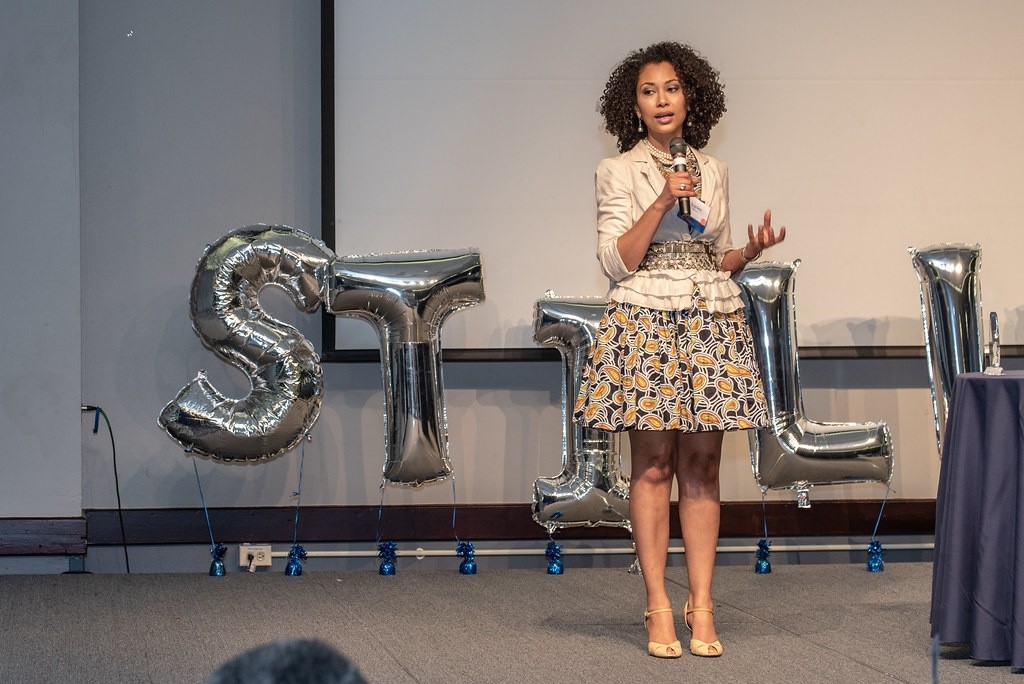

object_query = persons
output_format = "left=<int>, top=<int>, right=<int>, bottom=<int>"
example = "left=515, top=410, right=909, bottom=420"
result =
left=571, top=43, right=785, bottom=657
left=208, top=638, right=368, bottom=684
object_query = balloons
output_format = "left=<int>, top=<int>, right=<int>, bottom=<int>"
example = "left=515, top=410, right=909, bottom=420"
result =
left=156, top=224, right=986, bottom=529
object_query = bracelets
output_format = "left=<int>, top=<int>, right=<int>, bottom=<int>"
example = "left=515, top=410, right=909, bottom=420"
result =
left=741, top=248, right=763, bottom=262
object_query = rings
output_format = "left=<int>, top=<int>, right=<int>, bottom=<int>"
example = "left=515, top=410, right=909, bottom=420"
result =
left=680, top=184, right=686, bottom=191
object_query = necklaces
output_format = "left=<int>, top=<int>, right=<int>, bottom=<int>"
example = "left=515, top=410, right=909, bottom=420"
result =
left=643, top=137, right=674, bottom=165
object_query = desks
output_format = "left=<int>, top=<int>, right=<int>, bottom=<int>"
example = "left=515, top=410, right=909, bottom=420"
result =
left=929, top=368, right=1024, bottom=673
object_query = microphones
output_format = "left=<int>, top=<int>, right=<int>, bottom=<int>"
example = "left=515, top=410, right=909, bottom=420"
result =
left=670, top=137, right=691, bottom=219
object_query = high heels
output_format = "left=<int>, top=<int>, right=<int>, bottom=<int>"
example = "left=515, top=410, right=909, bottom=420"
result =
left=684, top=601, right=723, bottom=656
left=644, top=606, right=682, bottom=657
